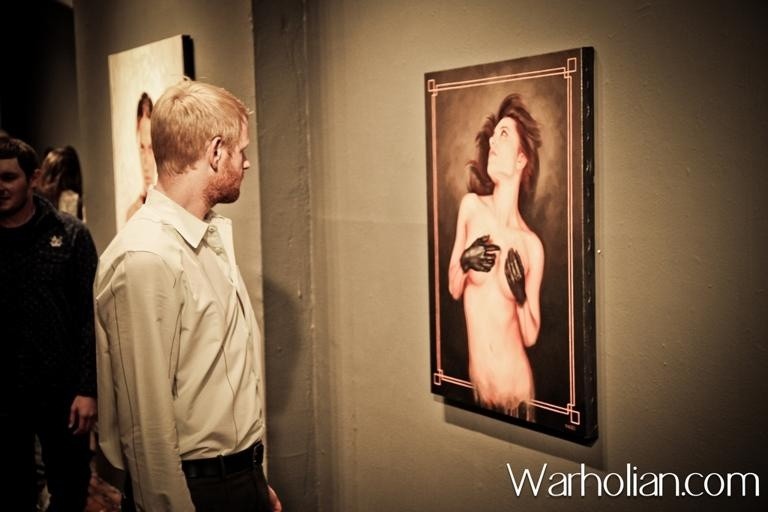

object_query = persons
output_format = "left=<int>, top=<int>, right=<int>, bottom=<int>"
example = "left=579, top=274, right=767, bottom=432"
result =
left=123, top=92, right=157, bottom=219
left=441, top=94, right=545, bottom=424
left=34, top=147, right=87, bottom=228
left=0, top=129, right=97, bottom=511
left=91, top=78, right=288, bottom=511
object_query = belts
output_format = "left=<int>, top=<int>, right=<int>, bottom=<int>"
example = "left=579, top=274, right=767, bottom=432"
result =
left=181, top=440, right=264, bottom=480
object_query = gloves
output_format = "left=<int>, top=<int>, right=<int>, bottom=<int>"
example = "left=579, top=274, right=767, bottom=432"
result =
left=504, top=247, right=527, bottom=307
left=459, top=234, right=501, bottom=273
